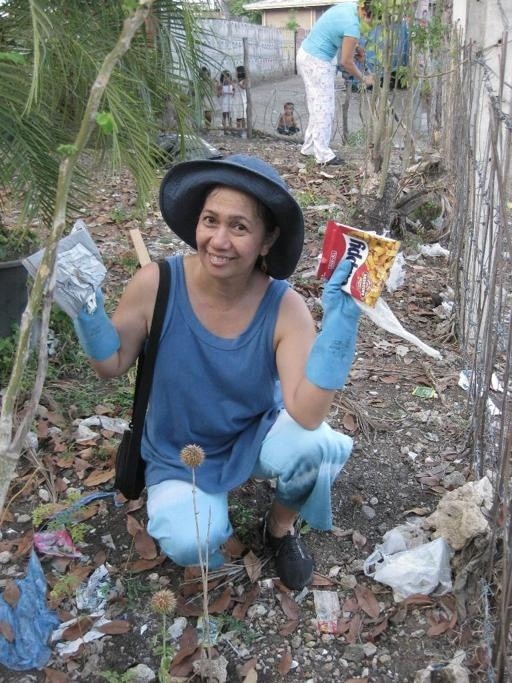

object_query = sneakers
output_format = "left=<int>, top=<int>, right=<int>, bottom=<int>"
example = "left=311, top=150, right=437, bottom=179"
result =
left=318, top=156, right=346, bottom=166
left=261, top=508, right=315, bottom=592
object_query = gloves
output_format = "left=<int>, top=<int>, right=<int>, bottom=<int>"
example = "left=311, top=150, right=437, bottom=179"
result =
left=73, top=285, right=123, bottom=362
left=304, top=258, right=362, bottom=392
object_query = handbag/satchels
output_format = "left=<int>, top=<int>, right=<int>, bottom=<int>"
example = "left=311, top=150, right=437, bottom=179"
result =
left=112, top=429, right=147, bottom=501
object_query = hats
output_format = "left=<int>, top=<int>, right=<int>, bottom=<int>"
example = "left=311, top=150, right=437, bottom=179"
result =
left=158, top=154, right=306, bottom=282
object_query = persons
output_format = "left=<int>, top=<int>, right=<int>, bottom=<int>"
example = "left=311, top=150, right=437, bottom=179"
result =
left=296, top=0, right=375, bottom=164
left=215, top=68, right=234, bottom=128
left=199, top=66, right=217, bottom=130
left=49, top=153, right=362, bottom=592
left=276, top=102, right=299, bottom=135
left=232, top=64, right=250, bottom=129
